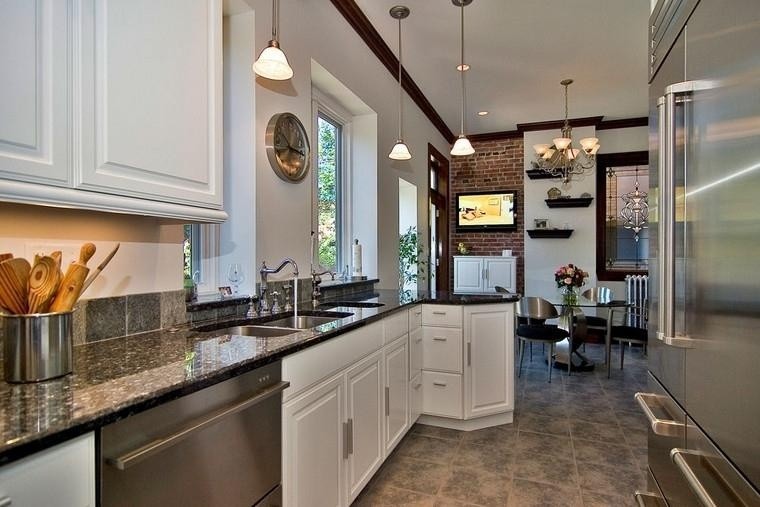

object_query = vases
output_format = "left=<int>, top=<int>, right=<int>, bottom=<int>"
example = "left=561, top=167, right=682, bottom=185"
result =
left=561, top=288, right=578, bottom=306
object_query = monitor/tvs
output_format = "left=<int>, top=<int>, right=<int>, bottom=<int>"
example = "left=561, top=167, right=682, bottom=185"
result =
left=455, top=190, right=518, bottom=234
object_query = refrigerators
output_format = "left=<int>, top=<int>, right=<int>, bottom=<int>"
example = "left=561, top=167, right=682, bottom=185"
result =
left=634, top=1, right=760, bottom=507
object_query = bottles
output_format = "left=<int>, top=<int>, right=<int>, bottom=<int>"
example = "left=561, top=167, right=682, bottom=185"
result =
left=351, top=239, right=362, bottom=276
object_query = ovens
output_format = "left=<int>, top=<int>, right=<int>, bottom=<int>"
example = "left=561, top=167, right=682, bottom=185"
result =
left=101, top=359, right=290, bottom=507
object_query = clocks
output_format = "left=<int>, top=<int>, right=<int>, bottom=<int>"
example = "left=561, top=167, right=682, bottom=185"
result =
left=265, top=111, right=312, bottom=184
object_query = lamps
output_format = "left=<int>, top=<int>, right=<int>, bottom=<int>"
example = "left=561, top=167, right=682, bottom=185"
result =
left=447, top=1, right=476, bottom=156
left=532, top=79, right=601, bottom=185
left=388, top=5, right=413, bottom=161
left=252, top=0, right=294, bottom=81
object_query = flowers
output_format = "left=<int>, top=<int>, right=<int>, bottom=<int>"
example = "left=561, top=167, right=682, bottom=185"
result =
left=554, top=263, right=590, bottom=292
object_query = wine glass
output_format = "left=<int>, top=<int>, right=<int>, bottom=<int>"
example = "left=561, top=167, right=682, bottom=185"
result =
left=227, top=263, right=244, bottom=295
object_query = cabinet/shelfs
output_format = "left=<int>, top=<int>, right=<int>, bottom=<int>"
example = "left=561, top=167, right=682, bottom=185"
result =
left=0, top=1, right=227, bottom=220
left=452, top=256, right=518, bottom=299
left=526, top=167, right=593, bottom=239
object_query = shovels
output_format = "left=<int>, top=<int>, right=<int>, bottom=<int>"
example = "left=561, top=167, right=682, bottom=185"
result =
left=0, top=257, right=32, bottom=314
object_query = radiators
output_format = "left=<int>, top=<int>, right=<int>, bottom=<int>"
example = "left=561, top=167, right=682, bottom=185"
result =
left=622, top=273, right=650, bottom=353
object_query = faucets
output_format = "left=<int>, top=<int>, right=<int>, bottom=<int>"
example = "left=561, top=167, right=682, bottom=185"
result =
left=258, top=256, right=299, bottom=289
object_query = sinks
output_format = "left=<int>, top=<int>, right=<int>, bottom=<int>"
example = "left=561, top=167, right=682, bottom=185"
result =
left=244, top=314, right=345, bottom=332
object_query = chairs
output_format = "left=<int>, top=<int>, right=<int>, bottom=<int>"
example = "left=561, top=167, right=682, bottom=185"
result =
left=582, top=287, right=615, bottom=352
left=513, top=297, right=572, bottom=383
left=596, top=298, right=648, bottom=370
